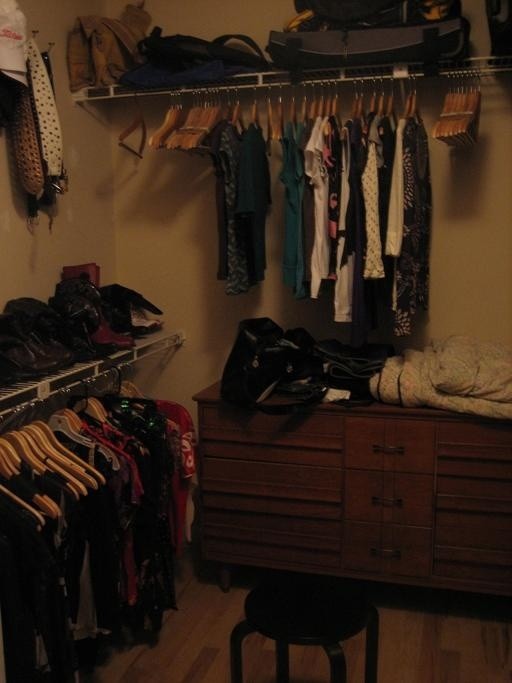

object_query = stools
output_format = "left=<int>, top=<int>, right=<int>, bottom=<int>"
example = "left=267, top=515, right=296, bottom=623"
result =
left=230, top=573, right=379, bottom=683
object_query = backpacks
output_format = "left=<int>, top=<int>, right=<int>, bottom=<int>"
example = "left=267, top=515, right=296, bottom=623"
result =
left=140, top=27, right=270, bottom=72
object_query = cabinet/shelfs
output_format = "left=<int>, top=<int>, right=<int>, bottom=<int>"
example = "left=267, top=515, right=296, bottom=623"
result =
left=342, top=407, right=435, bottom=577
left=197, top=403, right=345, bottom=569
left=431, top=416, right=512, bottom=585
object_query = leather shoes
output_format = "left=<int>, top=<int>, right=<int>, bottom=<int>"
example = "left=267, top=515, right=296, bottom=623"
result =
left=0, top=345, right=58, bottom=375
left=10, top=332, right=73, bottom=364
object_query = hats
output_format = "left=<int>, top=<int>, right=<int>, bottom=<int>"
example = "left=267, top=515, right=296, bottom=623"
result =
left=0, top=1, right=28, bottom=87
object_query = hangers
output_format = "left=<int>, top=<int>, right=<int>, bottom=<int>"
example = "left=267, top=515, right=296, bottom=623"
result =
left=117, top=92, right=146, bottom=158
left=432, top=70, right=481, bottom=149
left=148, top=73, right=423, bottom=156
left=0, top=362, right=149, bottom=531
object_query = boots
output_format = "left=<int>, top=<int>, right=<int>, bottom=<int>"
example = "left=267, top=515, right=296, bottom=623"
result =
left=63, top=262, right=135, bottom=350
